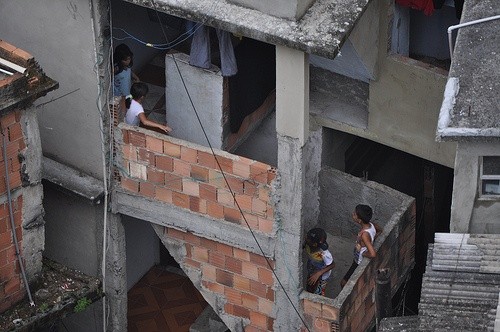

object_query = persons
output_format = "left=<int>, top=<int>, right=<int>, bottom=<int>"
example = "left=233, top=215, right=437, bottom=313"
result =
left=302, top=228, right=335, bottom=296
left=123, top=82, right=173, bottom=132
left=109, top=44, right=139, bottom=97
left=340, top=204, right=381, bottom=288
